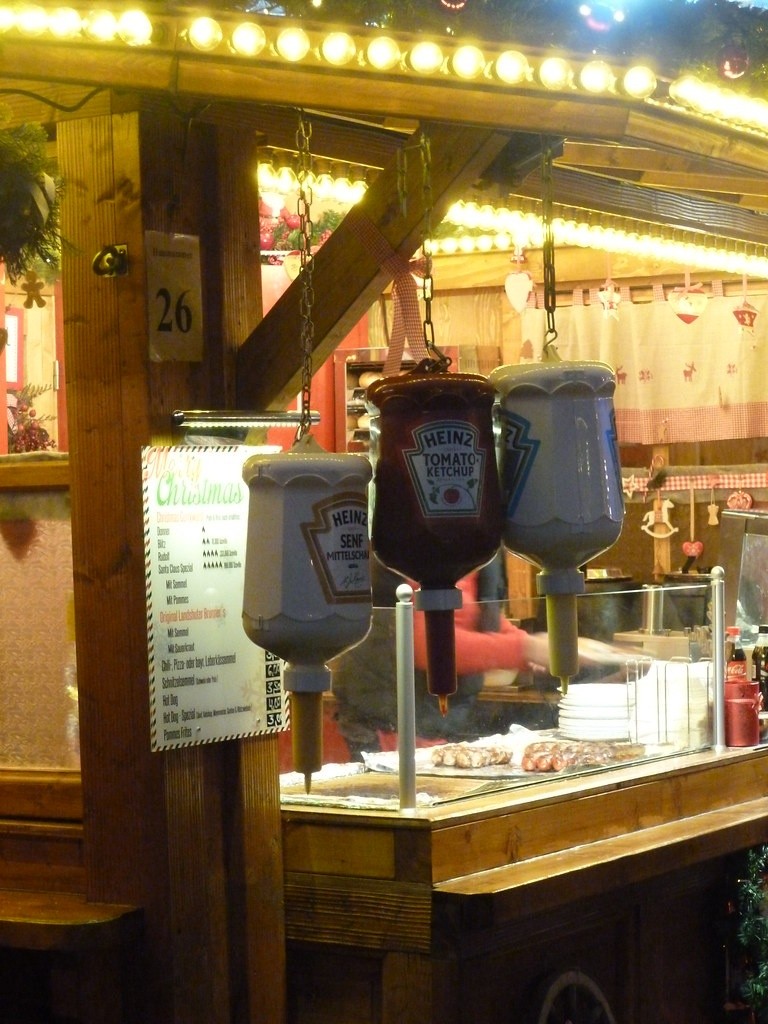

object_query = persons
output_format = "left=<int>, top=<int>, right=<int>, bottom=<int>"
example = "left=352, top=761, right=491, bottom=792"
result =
left=330, top=523, right=600, bottom=753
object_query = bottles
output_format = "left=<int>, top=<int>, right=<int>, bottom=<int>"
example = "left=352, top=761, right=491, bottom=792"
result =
left=361, top=372, right=504, bottom=612
left=726, top=626, right=747, bottom=682
left=751, top=625, right=768, bottom=713
left=491, top=360, right=627, bottom=598
left=238, top=452, right=375, bottom=694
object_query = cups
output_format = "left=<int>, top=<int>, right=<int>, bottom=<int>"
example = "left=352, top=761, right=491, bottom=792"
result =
left=724, top=681, right=760, bottom=747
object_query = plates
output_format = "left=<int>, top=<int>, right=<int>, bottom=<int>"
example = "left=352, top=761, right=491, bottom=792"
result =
left=556, top=683, right=637, bottom=742
